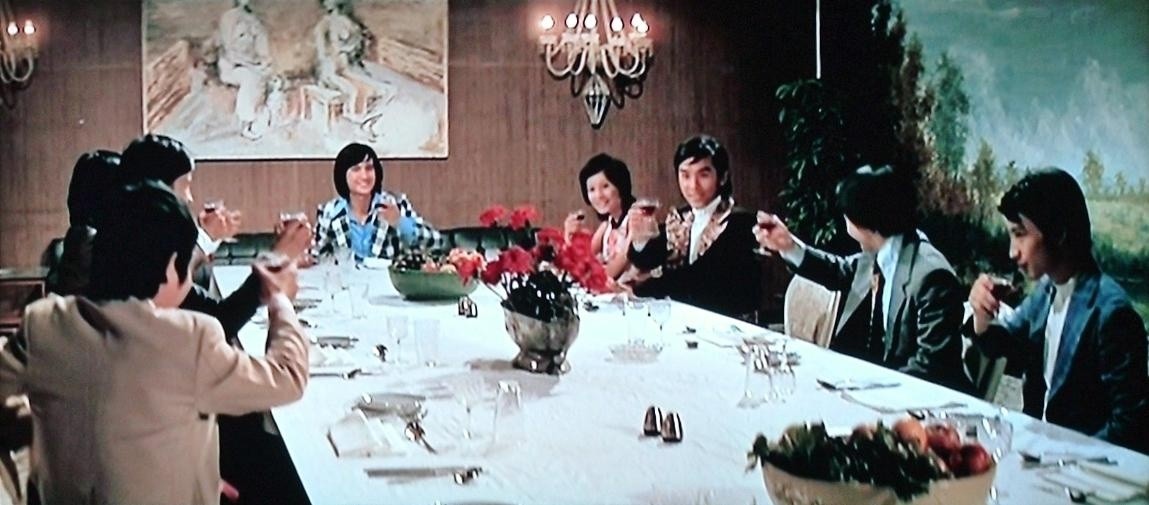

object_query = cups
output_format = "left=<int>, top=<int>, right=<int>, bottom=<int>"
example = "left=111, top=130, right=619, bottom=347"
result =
left=334, top=246, right=351, bottom=270
left=491, top=378, right=524, bottom=447
left=347, top=282, right=369, bottom=321
left=415, top=319, right=439, bottom=368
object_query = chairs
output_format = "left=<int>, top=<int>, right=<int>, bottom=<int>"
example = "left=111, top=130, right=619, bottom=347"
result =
left=974, top=300, right=1017, bottom=401
left=783, top=273, right=842, bottom=350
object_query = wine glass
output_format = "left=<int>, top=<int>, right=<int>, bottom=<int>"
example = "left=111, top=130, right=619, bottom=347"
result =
left=280, top=210, right=299, bottom=230
left=374, top=198, right=386, bottom=210
left=575, top=209, right=586, bottom=221
left=646, top=296, right=672, bottom=351
left=204, top=199, right=225, bottom=215
left=753, top=211, right=774, bottom=257
left=986, top=271, right=1014, bottom=323
left=766, top=337, right=796, bottom=402
left=258, top=254, right=290, bottom=304
left=386, top=315, right=408, bottom=367
left=321, top=257, right=334, bottom=274
left=323, top=271, right=342, bottom=315
left=637, top=197, right=660, bottom=238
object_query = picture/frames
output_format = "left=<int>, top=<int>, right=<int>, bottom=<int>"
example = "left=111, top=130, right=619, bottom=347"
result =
left=141, top=0, right=451, bottom=160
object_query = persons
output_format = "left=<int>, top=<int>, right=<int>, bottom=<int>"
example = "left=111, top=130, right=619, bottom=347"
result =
left=752, top=161, right=967, bottom=391
left=627, top=134, right=759, bottom=317
left=565, top=153, right=660, bottom=294
left=0, top=178, right=310, bottom=505
left=41, top=149, right=240, bottom=292
left=961, top=169, right=1148, bottom=505
left=64, top=134, right=315, bottom=345
left=295, top=143, right=447, bottom=268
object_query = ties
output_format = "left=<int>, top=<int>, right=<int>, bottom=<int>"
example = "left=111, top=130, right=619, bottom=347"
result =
left=868, top=273, right=885, bottom=364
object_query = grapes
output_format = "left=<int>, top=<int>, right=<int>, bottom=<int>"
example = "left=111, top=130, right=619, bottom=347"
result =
left=848, top=445, right=937, bottom=503
left=397, top=247, right=444, bottom=272
left=751, top=419, right=844, bottom=486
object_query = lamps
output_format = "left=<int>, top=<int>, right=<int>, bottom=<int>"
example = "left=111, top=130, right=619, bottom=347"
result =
left=0, top=1, right=39, bottom=84
left=529, top=0, right=654, bottom=128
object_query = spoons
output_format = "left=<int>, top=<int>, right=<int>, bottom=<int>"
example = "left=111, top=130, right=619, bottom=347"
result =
left=1015, top=448, right=1108, bottom=467
left=310, top=368, right=362, bottom=381
left=1064, top=487, right=1087, bottom=504
left=905, top=408, right=923, bottom=419
left=815, top=378, right=901, bottom=392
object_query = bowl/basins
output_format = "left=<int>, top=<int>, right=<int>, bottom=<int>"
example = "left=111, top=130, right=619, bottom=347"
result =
left=387, top=266, right=477, bottom=301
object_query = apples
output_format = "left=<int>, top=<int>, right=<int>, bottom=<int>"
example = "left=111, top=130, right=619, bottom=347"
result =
left=953, top=445, right=986, bottom=473
left=926, top=423, right=961, bottom=456
left=444, top=246, right=476, bottom=274
left=850, top=425, right=873, bottom=444
left=894, top=417, right=926, bottom=450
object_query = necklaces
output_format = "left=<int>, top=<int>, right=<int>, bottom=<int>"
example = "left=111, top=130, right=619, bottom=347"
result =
left=604, top=214, right=629, bottom=262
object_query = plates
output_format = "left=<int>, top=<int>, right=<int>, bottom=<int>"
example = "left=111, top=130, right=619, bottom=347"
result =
left=758, top=437, right=996, bottom=504
